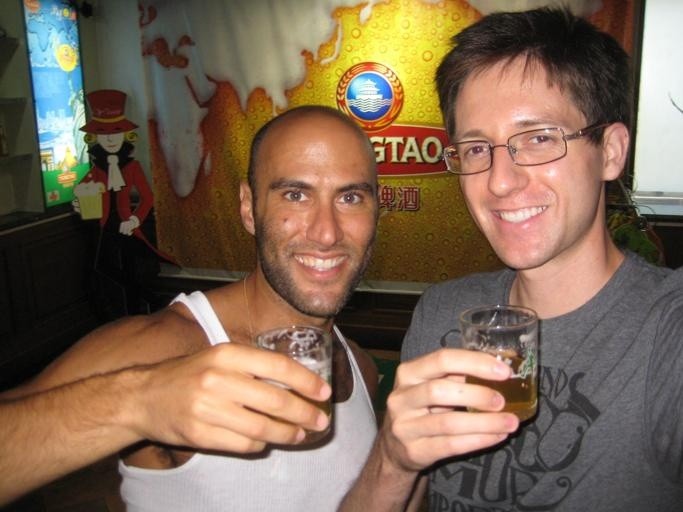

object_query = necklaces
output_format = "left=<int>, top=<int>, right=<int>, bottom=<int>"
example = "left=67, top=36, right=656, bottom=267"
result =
left=243, top=271, right=254, bottom=345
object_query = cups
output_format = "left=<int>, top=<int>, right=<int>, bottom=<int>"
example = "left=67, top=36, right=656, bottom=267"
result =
left=254, top=323, right=334, bottom=450
left=459, top=302, right=540, bottom=424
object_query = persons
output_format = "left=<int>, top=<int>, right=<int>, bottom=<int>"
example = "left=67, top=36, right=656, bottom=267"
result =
left=338, top=4, right=683, bottom=512
left=73, top=87, right=183, bottom=324
left=0, top=104, right=379, bottom=512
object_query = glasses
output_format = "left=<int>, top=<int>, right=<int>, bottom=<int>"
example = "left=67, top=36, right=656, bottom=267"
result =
left=437, top=121, right=614, bottom=175
